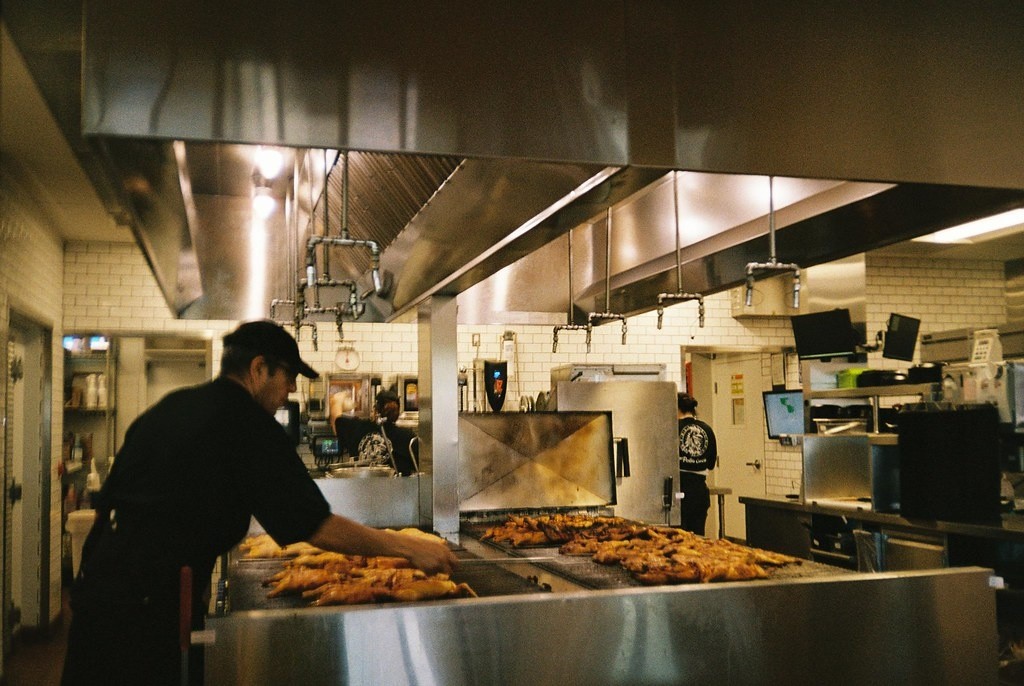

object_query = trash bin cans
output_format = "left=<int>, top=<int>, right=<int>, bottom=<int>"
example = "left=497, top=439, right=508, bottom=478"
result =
left=65, top=508, right=97, bottom=580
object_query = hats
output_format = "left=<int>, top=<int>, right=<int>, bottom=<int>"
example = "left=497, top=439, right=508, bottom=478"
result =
left=224, top=321, right=319, bottom=379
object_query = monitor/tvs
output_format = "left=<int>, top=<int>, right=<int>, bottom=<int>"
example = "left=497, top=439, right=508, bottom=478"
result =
left=762, top=389, right=804, bottom=440
left=313, top=435, right=340, bottom=472
left=790, top=309, right=856, bottom=360
left=882, top=312, right=921, bottom=362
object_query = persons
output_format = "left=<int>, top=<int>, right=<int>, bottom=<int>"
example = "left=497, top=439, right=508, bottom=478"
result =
left=677, top=391, right=717, bottom=537
left=330, top=390, right=419, bottom=478
left=59, top=321, right=462, bottom=686
